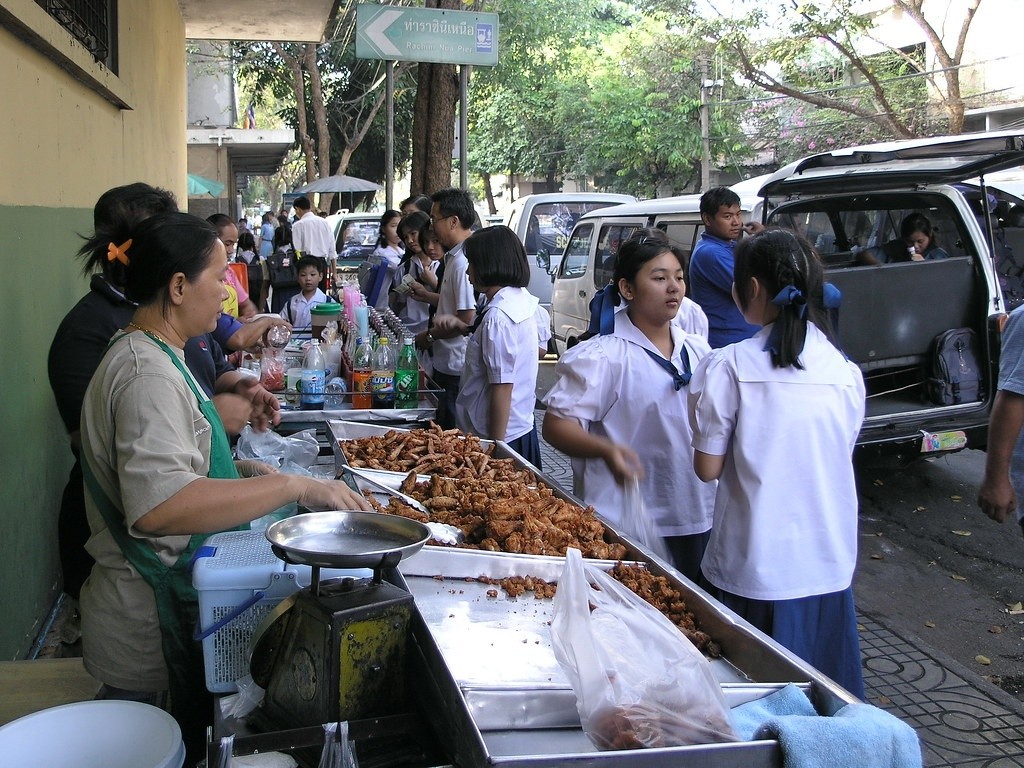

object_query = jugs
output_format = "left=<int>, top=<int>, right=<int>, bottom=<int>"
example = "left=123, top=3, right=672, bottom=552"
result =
left=310, top=303, right=342, bottom=339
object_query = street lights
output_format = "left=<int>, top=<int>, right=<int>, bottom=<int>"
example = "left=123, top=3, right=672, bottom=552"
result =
left=698, top=78, right=724, bottom=195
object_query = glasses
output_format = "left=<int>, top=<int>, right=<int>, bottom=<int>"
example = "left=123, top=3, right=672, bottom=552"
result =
left=430, top=214, right=457, bottom=226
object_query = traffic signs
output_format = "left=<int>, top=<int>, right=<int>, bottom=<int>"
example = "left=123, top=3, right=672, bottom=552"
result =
left=355, top=1, right=500, bottom=66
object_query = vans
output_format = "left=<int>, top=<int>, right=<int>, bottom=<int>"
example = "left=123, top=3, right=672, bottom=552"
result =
left=497, top=193, right=639, bottom=356
left=326, top=213, right=382, bottom=301
left=536, top=128, right=1024, bottom=474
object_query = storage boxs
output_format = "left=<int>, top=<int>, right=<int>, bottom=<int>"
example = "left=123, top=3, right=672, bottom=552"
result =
left=192, top=529, right=374, bottom=693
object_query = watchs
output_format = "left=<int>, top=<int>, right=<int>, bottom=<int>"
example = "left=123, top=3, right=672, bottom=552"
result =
left=425, top=328, right=436, bottom=344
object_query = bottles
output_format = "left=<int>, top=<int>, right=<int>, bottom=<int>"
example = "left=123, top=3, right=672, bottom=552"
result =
left=326, top=376, right=347, bottom=406
left=301, top=339, right=324, bottom=411
left=341, top=306, right=418, bottom=408
left=265, top=325, right=290, bottom=353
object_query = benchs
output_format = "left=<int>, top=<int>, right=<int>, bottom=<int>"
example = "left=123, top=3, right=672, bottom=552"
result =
left=817, top=257, right=981, bottom=371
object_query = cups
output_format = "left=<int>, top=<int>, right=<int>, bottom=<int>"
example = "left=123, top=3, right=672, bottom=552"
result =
left=287, top=368, right=301, bottom=407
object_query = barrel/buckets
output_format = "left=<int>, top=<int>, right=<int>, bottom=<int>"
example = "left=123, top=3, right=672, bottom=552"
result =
left=1, top=701, right=184, bottom=768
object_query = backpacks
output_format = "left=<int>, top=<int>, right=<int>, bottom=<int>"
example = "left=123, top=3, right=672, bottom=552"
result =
left=238, top=254, right=264, bottom=303
left=266, top=251, right=307, bottom=290
left=925, top=328, right=987, bottom=405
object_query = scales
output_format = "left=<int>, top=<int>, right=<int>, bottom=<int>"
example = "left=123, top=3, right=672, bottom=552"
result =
left=247, top=511, right=429, bottom=764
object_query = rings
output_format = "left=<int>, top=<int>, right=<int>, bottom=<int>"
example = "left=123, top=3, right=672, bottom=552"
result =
left=286, top=319, right=289, bottom=323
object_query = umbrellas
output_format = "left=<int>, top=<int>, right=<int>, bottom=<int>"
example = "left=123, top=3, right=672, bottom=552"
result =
left=303, top=173, right=384, bottom=210
left=187, top=173, right=227, bottom=198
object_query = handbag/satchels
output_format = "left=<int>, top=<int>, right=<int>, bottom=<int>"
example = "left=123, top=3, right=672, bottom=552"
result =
left=548, top=547, right=741, bottom=750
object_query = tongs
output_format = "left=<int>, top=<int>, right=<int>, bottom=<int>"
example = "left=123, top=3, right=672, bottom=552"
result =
left=424, top=522, right=466, bottom=545
left=334, top=465, right=433, bottom=515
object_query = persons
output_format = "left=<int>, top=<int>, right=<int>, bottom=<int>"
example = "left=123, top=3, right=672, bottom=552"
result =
left=850, top=222, right=908, bottom=267
left=456, top=225, right=543, bottom=473
left=368, top=189, right=488, bottom=431
left=689, top=187, right=768, bottom=350
left=292, top=196, right=338, bottom=294
left=79, top=213, right=375, bottom=768
left=686, top=227, right=866, bottom=703
left=432, top=289, right=553, bottom=359
left=892, top=212, right=949, bottom=263
left=542, top=226, right=719, bottom=581
left=279, top=254, right=339, bottom=327
left=977, top=304, right=1024, bottom=530
left=779, top=213, right=804, bottom=234
left=47, top=183, right=294, bottom=603
